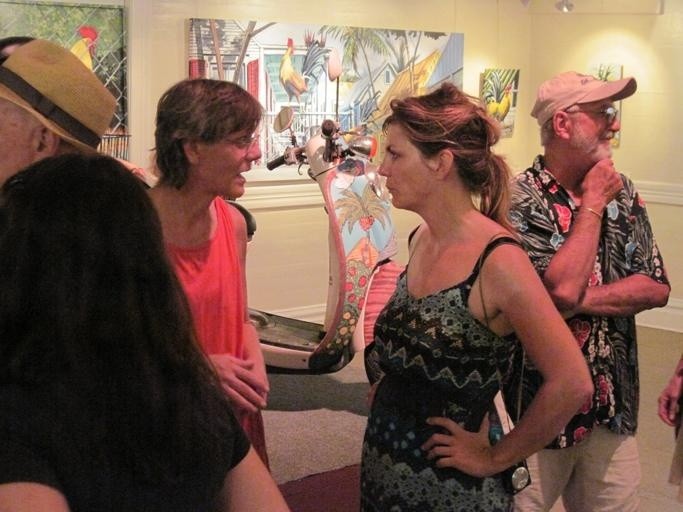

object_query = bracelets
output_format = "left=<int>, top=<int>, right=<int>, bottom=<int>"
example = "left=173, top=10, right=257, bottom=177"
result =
left=578, top=205, right=604, bottom=219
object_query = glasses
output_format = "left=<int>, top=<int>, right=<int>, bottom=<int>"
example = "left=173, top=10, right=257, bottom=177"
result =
left=219, top=133, right=261, bottom=148
left=566, top=105, right=619, bottom=122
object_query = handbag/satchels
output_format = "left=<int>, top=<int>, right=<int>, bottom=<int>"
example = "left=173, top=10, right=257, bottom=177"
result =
left=502, top=458, right=532, bottom=496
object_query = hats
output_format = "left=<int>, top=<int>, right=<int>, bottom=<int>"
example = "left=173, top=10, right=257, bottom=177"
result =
left=0, top=39, right=117, bottom=155
left=530, top=70, right=638, bottom=127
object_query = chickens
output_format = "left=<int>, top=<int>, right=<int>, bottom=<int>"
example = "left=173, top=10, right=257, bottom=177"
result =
left=482, top=71, right=512, bottom=124
left=68, top=24, right=100, bottom=70
left=278, top=36, right=331, bottom=102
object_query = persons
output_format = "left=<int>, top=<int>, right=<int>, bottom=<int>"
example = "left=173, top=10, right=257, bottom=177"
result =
left=0, top=36, right=115, bottom=184
left=1, top=152, right=290, bottom=511
left=501, top=70, right=671, bottom=512
left=659, top=352, right=683, bottom=504
left=360, top=81, right=594, bottom=512
left=149, top=80, right=269, bottom=471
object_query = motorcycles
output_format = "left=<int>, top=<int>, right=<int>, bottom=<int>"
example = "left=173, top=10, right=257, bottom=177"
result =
left=225, top=105, right=407, bottom=378
